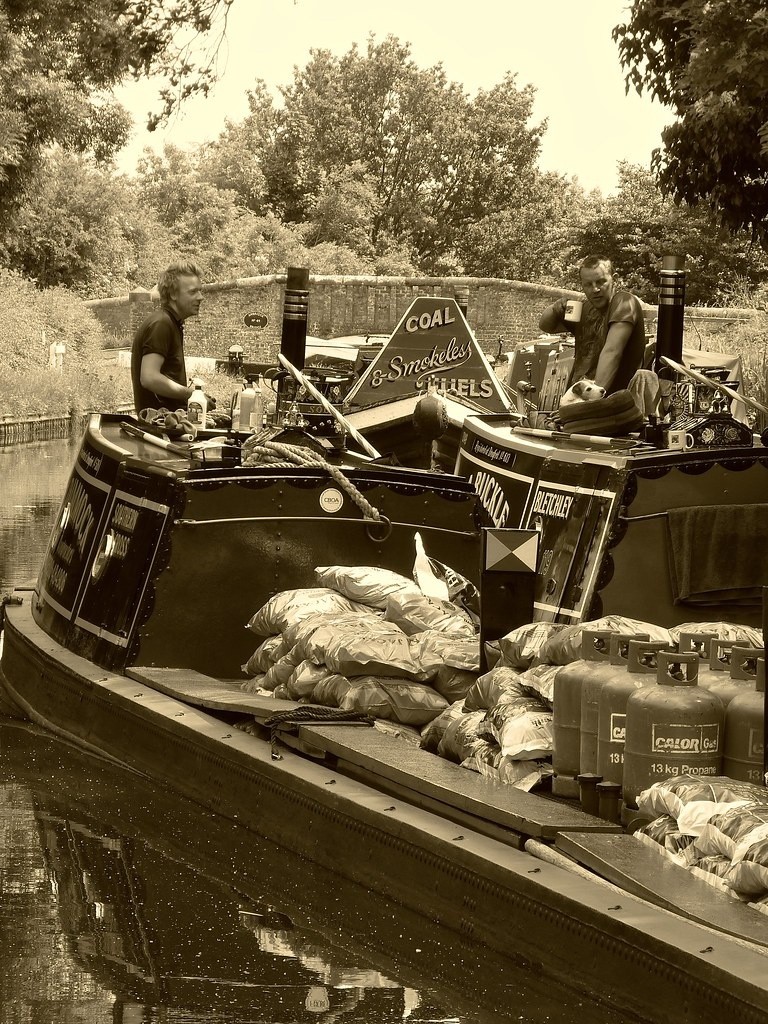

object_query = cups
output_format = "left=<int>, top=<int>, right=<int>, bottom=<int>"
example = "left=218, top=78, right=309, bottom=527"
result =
left=669, top=431, right=694, bottom=450
left=564, top=301, right=582, bottom=322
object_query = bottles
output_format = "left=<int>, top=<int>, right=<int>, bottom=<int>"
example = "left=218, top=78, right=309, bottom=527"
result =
left=250, top=392, right=263, bottom=427
left=239, top=385, right=255, bottom=431
left=187, top=386, right=206, bottom=430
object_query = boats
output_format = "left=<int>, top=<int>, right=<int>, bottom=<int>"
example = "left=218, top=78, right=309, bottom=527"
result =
left=0, top=252, right=768, bottom=1024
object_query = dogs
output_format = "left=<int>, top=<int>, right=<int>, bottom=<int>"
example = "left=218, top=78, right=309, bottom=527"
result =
left=560, top=376, right=606, bottom=407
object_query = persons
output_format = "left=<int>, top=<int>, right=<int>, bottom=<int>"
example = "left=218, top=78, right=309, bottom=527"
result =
left=131, top=260, right=204, bottom=417
left=539, top=254, right=646, bottom=424
